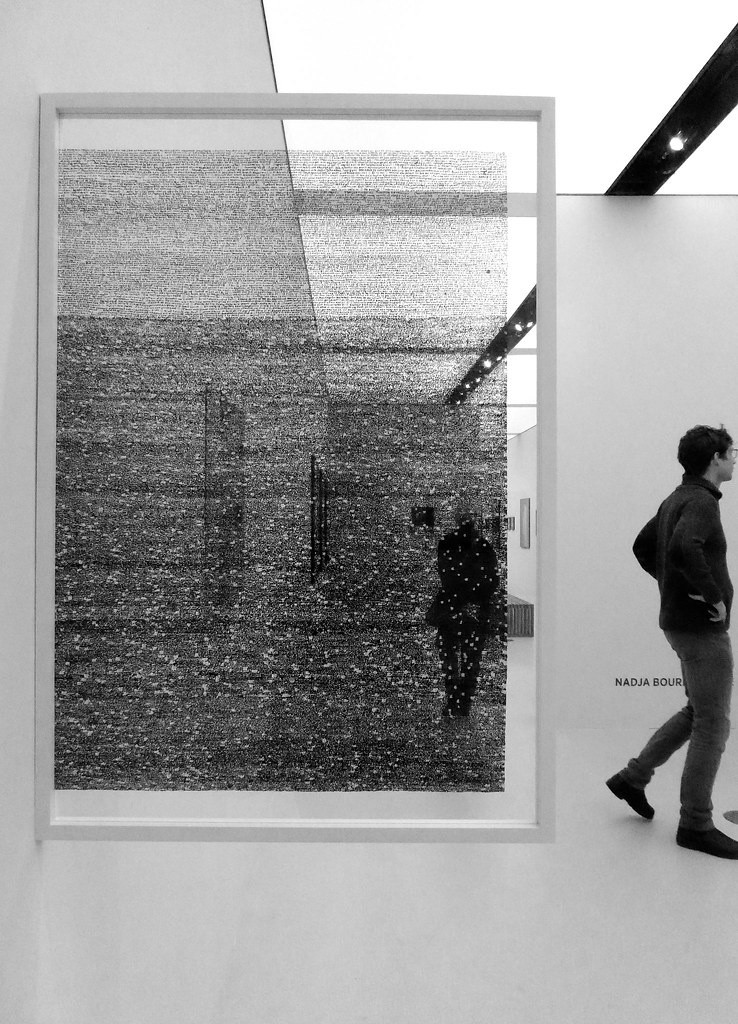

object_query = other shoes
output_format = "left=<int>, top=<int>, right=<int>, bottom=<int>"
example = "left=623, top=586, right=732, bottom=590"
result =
left=606, top=772, right=655, bottom=819
left=442, top=700, right=455, bottom=715
left=459, top=701, right=470, bottom=715
left=676, top=826, right=738, bottom=859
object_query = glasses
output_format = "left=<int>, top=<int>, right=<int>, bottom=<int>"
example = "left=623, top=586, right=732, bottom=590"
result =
left=729, top=448, right=738, bottom=459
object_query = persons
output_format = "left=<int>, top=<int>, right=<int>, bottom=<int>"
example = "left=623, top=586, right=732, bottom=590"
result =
left=425, top=500, right=500, bottom=720
left=606, top=424, right=738, bottom=859
